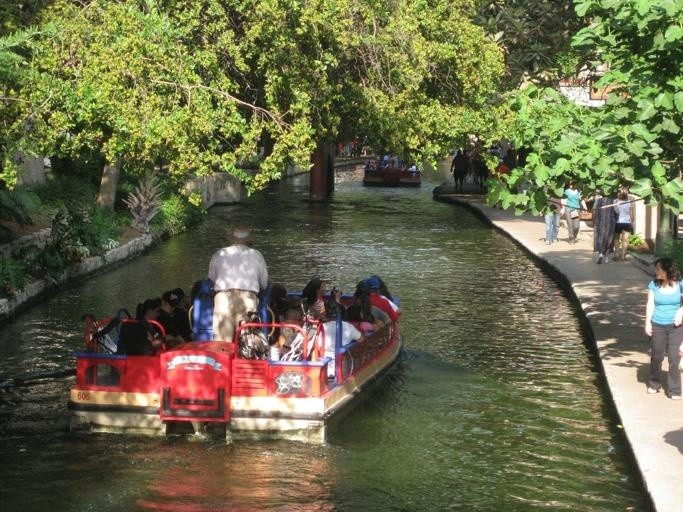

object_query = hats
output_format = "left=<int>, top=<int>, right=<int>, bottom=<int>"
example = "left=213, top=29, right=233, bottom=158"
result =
left=224, top=226, right=256, bottom=243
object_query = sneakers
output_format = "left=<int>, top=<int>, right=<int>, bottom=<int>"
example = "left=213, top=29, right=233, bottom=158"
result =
left=596, top=253, right=625, bottom=265
left=671, top=396, right=680, bottom=400
left=647, top=386, right=657, bottom=394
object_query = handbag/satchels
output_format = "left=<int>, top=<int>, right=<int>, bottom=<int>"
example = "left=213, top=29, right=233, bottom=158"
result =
left=571, top=210, right=578, bottom=219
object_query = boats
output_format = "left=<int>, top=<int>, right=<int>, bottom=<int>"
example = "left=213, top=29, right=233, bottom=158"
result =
left=64, top=275, right=408, bottom=446
left=360, top=155, right=422, bottom=188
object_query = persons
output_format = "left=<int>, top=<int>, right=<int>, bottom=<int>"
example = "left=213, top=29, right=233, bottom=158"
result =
left=542, top=204, right=562, bottom=246
left=121, top=275, right=387, bottom=377
left=678, top=340, right=683, bottom=359
left=206, top=225, right=268, bottom=343
left=591, top=185, right=635, bottom=265
left=336, top=138, right=419, bottom=175
left=560, top=179, right=589, bottom=244
left=643, top=257, right=682, bottom=400
left=446, top=148, right=534, bottom=196
